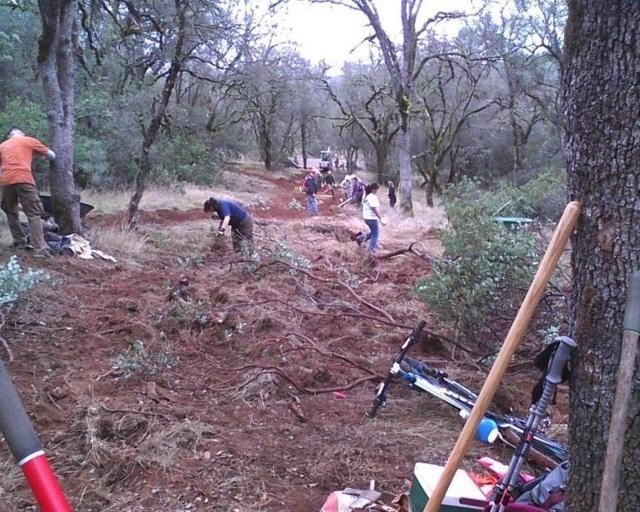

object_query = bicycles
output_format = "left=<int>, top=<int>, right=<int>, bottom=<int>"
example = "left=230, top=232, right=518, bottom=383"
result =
left=369, top=320, right=569, bottom=470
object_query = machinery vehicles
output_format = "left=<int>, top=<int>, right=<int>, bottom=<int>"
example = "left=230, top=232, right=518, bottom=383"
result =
left=319, top=151, right=333, bottom=173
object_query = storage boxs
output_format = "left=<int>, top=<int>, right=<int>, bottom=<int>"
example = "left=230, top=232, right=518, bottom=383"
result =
left=408, top=462, right=489, bottom=511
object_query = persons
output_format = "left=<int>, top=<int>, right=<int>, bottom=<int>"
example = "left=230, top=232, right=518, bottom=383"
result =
left=353, top=181, right=387, bottom=253
left=202, top=197, right=254, bottom=257
left=303, top=166, right=398, bottom=216
left=0, top=125, right=58, bottom=251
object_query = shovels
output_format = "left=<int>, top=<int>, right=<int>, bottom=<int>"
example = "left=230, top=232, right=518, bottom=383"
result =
left=329, top=197, right=353, bottom=212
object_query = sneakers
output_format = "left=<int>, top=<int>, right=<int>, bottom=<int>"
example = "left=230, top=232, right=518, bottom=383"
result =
left=33, top=250, right=54, bottom=259
